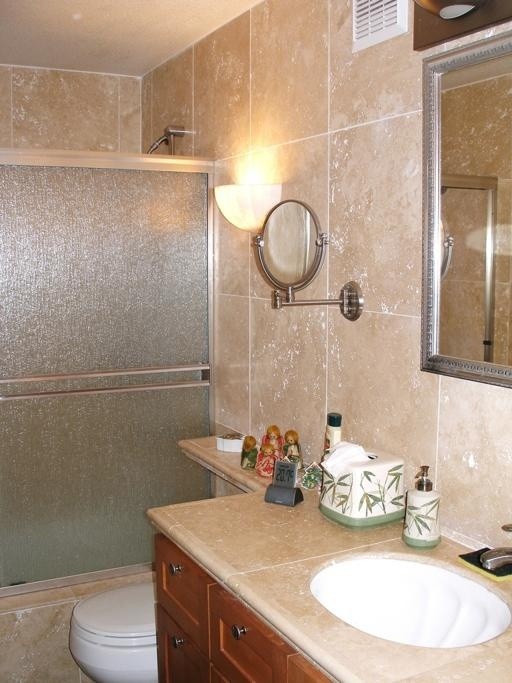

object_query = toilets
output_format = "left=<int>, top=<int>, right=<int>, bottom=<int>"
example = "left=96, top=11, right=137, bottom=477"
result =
left=68, top=582, right=158, bottom=682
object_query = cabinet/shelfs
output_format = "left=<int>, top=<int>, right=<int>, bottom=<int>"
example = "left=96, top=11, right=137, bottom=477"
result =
left=146, top=532, right=328, bottom=683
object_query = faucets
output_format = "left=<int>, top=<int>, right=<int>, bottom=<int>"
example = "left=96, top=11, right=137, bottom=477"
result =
left=480, top=523, right=512, bottom=572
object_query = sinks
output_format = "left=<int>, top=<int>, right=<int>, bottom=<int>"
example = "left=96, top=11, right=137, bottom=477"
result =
left=309, top=551, right=512, bottom=650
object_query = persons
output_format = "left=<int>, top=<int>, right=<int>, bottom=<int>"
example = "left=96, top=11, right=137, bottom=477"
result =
left=262, top=425, right=283, bottom=460
left=281, top=430, right=303, bottom=469
left=256, top=443, right=278, bottom=477
left=241, top=436, right=258, bottom=469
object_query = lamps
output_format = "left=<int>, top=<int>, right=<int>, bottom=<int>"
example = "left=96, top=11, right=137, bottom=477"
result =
left=214, top=161, right=283, bottom=234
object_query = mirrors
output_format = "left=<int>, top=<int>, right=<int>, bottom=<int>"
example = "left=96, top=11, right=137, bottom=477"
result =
left=260, top=201, right=323, bottom=291
left=419, top=30, right=512, bottom=388
